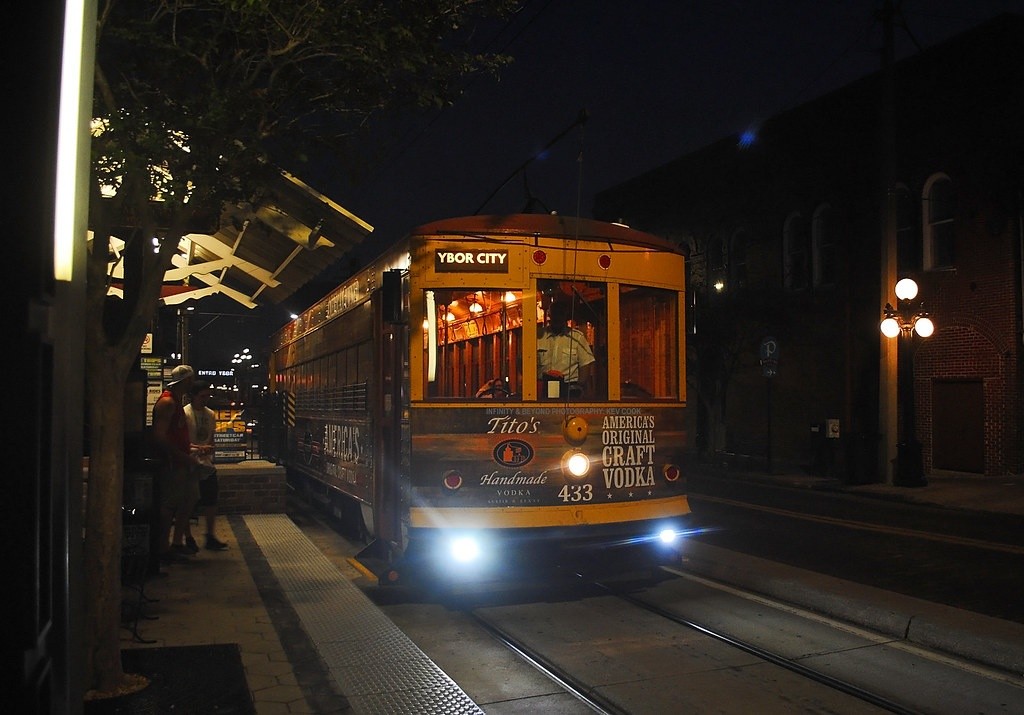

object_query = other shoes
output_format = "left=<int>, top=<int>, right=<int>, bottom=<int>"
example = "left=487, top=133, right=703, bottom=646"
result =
left=205, top=538, right=231, bottom=550
left=171, top=544, right=196, bottom=555
left=186, top=539, right=200, bottom=552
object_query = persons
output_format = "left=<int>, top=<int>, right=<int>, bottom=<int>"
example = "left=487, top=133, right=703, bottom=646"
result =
left=182, top=381, right=228, bottom=552
left=493, top=379, right=504, bottom=399
left=148, top=364, right=199, bottom=564
left=517, top=301, right=595, bottom=400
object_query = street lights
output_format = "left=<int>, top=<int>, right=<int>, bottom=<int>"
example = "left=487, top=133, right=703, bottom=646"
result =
left=879, top=278, right=934, bottom=487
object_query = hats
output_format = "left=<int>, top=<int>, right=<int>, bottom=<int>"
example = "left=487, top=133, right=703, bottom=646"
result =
left=167, top=364, right=194, bottom=386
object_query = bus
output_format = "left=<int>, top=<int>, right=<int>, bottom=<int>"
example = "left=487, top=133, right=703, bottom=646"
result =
left=266, top=211, right=693, bottom=599
